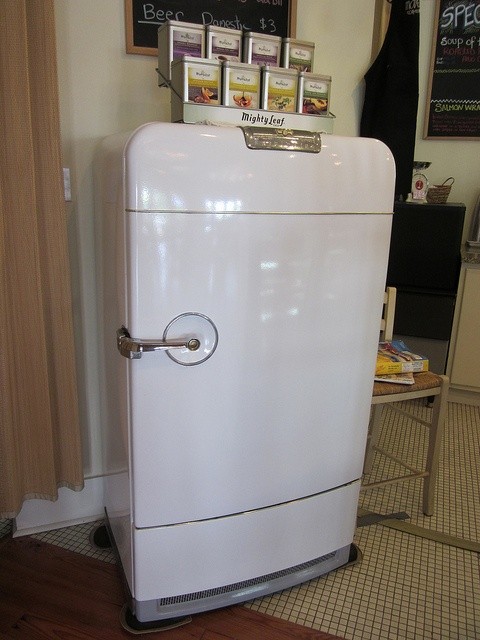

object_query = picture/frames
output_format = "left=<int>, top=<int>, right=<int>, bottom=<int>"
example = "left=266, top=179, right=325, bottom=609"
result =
left=123, top=0, right=299, bottom=58
left=423, top=0, right=479, bottom=141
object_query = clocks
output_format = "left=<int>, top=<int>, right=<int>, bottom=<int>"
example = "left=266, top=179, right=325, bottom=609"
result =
left=412, top=172, right=431, bottom=201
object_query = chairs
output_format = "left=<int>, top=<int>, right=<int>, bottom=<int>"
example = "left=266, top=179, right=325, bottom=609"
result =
left=358, top=285, right=449, bottom=516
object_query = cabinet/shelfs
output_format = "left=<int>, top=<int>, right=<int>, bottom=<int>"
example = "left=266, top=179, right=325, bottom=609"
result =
left=381, top=201, right=467, bottom=341
left=448, top=265, right=480, bottom=405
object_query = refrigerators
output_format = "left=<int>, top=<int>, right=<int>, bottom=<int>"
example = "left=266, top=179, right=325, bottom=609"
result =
left=96, top=120, right=396, bottom=623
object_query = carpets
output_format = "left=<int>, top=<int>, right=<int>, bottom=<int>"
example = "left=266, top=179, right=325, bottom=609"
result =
left=0, top=532, right=353, bottom=638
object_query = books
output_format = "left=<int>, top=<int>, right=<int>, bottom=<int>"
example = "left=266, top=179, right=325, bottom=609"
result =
left=374, top=336, right=429, bottom=375
left=374, top=373, right=415, bottom=385
